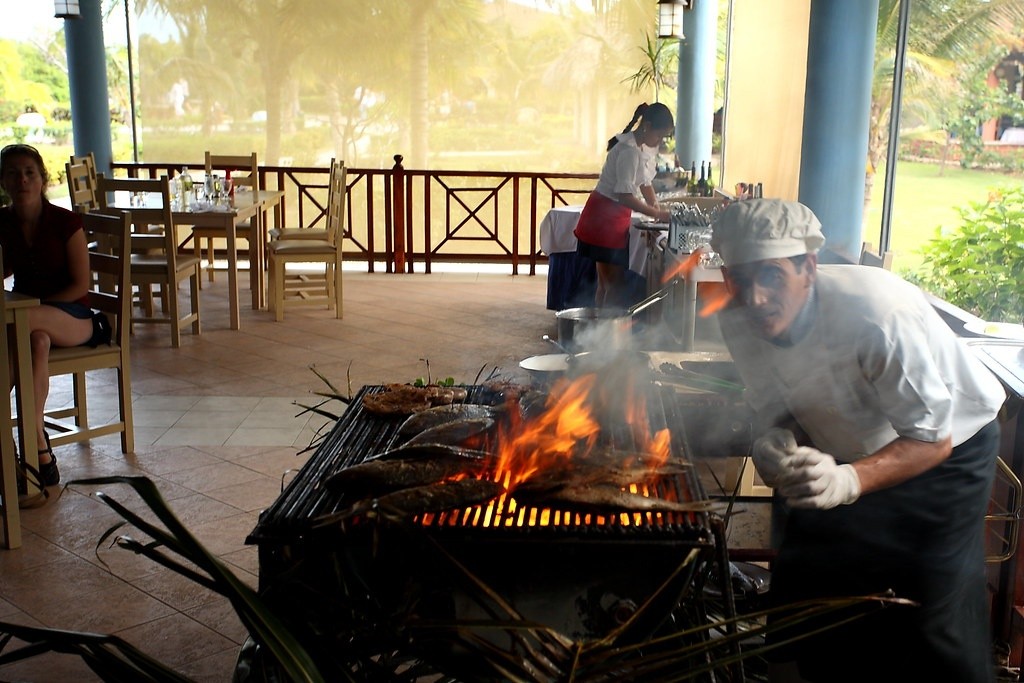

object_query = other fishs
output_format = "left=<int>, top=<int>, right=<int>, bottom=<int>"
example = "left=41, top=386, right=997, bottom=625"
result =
left=326, top=397, right=728, bottom=524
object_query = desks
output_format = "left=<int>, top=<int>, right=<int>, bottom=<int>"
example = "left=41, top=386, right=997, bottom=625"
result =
left=88, top=186, right=287, bottom=330
left=4, top=289, right=42, bottom=497
left=538, top=204, right=728, bottom=355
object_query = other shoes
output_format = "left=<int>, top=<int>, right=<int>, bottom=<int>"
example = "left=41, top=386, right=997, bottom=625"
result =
left=38, top=429, right=60, bottom=486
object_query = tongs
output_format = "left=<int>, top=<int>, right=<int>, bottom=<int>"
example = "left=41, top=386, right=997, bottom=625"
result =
left=650, top=362, right=747, bottom=396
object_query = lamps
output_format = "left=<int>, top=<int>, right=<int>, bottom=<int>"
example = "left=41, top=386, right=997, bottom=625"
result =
left=655, top=0, right=693, bottom=40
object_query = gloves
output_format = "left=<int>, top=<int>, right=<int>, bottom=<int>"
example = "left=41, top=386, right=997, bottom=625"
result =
left=751, top=427, right=798, bottom=487
left=781, top=446, right=862, bottom=509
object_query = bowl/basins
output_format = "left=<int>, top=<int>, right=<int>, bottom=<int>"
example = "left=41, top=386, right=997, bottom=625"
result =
left=652, top=170, right=694, bottom=193
left=518, top=354, right=571, bottom=383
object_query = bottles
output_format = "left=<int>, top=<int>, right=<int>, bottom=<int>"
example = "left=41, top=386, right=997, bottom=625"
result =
left=747, top=184, right=753, bottom=200
left=705, top=162, right=714, bottom=197
left=687, top=161, right=698, bottom=197
left=698, top=161, right=706, bottom=197
left=180, top=166, right=192, bottom=206
left=205, top=175, right=221, bottom=196
left=224, top=168, right=233, bottom=196
left=720, top=196, right=729, bottom=213
left=673, top=154, right=685, bottom=171
left=757, top=183, right=762, bottom=198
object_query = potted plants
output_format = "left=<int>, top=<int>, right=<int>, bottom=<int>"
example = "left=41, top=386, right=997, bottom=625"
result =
left=617, top=28, right=689, bottom=156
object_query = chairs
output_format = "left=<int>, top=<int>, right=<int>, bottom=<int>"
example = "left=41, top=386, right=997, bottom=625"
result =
left=266, top=156, right=350, bottom=321
left=192, top=151, right=259, bottom=290
left=13, top=203, right=136, bottom=455
left=97, top=171, right=203, bottom=351
left=63, top=151, right=152, bottom=310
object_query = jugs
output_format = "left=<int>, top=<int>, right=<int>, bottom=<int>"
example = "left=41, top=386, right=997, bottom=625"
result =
left=685, top=229, right=723, bottom=269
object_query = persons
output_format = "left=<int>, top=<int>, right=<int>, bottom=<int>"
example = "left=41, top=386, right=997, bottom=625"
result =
left=572, top=102, right=677, bottom=308
left=0, top=144, right=94, bottom=486
left=707, top=198, right=1007, bottom=683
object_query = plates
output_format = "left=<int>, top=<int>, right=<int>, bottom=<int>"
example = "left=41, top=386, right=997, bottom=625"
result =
left=963, top=322, right=1024, bottom=340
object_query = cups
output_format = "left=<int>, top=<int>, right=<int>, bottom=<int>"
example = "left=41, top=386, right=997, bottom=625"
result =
left=192, top=184, right=204, bottom=202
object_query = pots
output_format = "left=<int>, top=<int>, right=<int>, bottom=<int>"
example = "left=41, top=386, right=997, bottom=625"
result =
left=555, top=292, right=669, bottom=355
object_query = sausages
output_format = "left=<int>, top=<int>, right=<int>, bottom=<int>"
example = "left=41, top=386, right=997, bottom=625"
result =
left=362, top=383, right=468, bottom=413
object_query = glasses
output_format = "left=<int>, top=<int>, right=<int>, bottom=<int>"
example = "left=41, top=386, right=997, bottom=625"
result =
left=1, top=144, right=41, bottom=160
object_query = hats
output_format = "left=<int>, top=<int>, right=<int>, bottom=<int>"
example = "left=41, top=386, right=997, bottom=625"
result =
left=714, top=198, right=825, bottom=265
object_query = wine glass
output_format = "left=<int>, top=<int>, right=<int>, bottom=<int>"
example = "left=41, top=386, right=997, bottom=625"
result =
left=204, top=177, right=215, bottom=204
left=220, top=179, right=232, bottom=209
